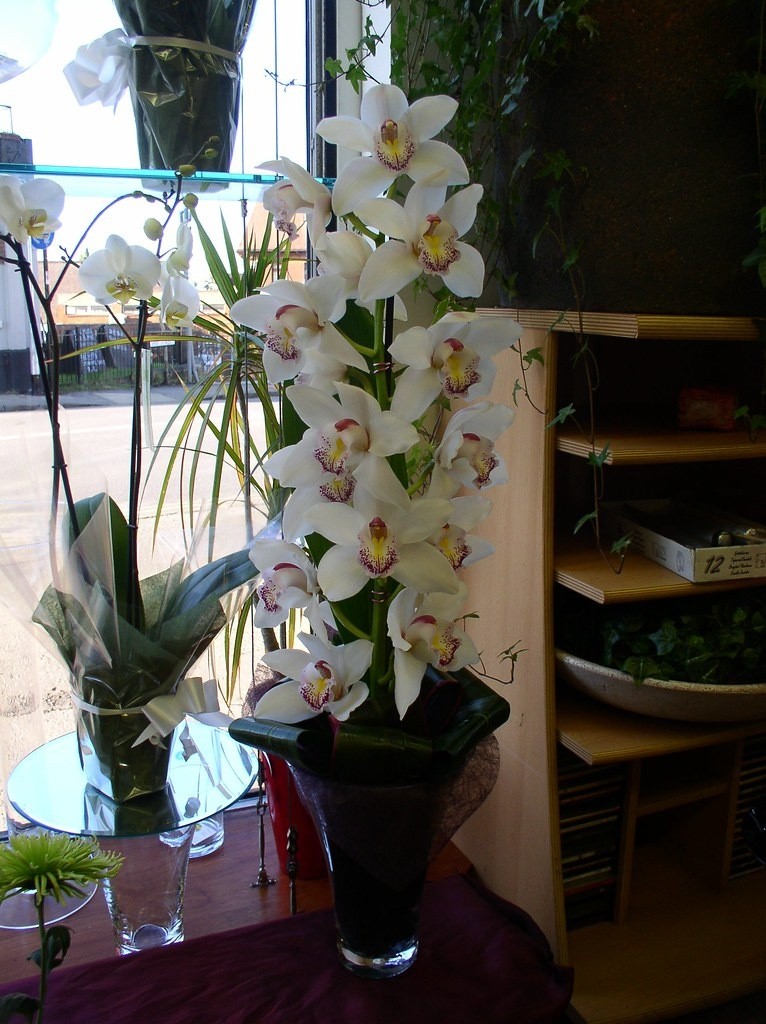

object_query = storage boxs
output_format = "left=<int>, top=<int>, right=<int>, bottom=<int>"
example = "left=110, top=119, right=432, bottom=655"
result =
left=599, top=498, right=766, bottom=584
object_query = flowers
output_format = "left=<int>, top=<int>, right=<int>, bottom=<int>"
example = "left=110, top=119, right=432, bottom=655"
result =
left=227, top=83, right=524, bottom=732
left=0, top=830, right=126, bottom=1024
left=0, top=129, right=202, bottom=803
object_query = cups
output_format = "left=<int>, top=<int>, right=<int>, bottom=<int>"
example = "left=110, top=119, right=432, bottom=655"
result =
left=87, top=824, right=196, bottom=956
left=158, top=811, right=225, bottom=859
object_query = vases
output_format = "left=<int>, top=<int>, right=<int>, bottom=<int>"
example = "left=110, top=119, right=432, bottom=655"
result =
left=230, top=667, right=510, bottom=985
left=86, top=820, right=197, bottom=955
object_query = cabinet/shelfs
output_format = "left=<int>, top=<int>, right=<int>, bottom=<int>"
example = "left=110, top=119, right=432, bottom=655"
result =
left=391, top=307, right=766, bottom=971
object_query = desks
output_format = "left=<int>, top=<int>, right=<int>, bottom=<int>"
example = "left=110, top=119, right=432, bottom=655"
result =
left=0, top=805, right=585, bottom=1024
